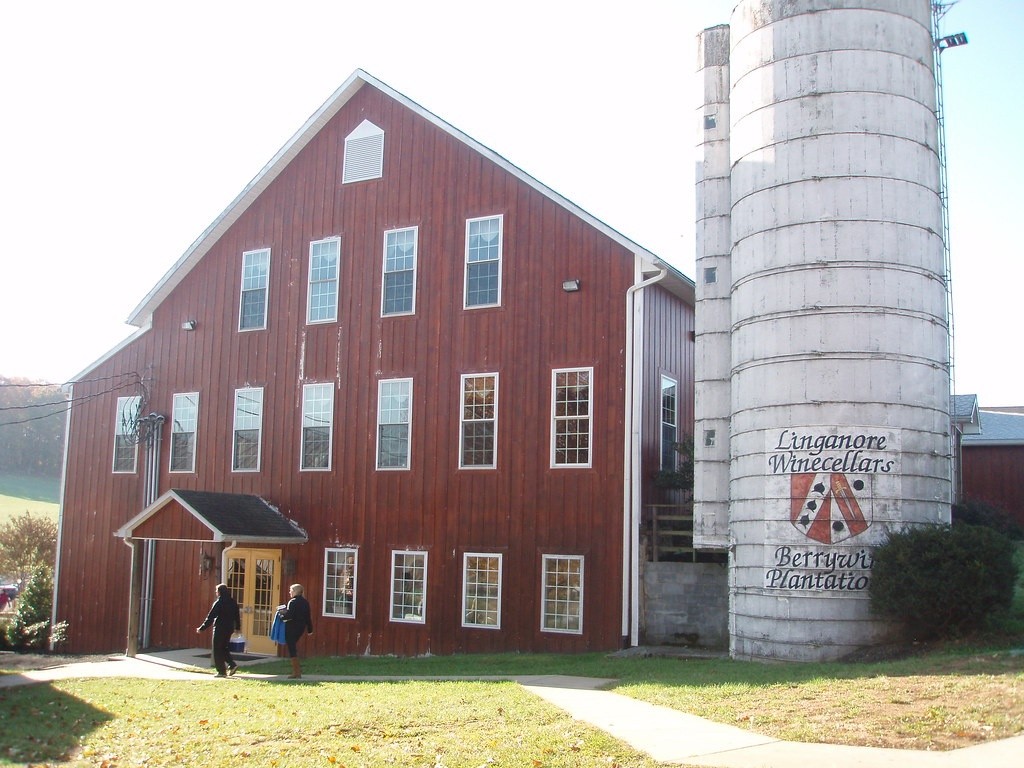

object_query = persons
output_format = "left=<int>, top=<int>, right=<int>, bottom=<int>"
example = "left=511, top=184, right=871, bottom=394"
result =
left=278, top=584, right=313, bottom=678
left=196, top=583, right=241, bottom=677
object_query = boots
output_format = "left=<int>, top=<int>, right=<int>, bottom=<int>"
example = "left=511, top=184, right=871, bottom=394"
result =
left=289, top=657, right=301, bottom=679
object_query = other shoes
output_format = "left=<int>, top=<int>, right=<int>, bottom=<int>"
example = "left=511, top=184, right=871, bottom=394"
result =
left=228, top=665, right=239, bottom=676
left=214, top=672, right=227, bottom=677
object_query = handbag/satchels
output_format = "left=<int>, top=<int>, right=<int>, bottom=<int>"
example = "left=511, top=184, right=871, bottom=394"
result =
left=269, top=611, right=279, bottom=641
left=278, top=617, right=286, bottom=644
